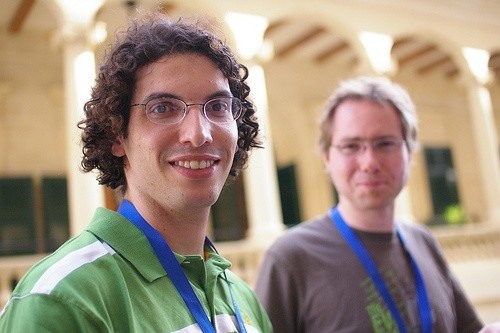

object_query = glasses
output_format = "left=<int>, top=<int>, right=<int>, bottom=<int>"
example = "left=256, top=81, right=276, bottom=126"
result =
left=127, top=96, right=247, bottom=124
left=327, top=137, right=409, bottom=156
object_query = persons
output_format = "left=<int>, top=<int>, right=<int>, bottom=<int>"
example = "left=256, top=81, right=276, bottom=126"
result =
left=1, top=6, right=278, bottom=333
left=254, top=75, right=500, bottom=333
